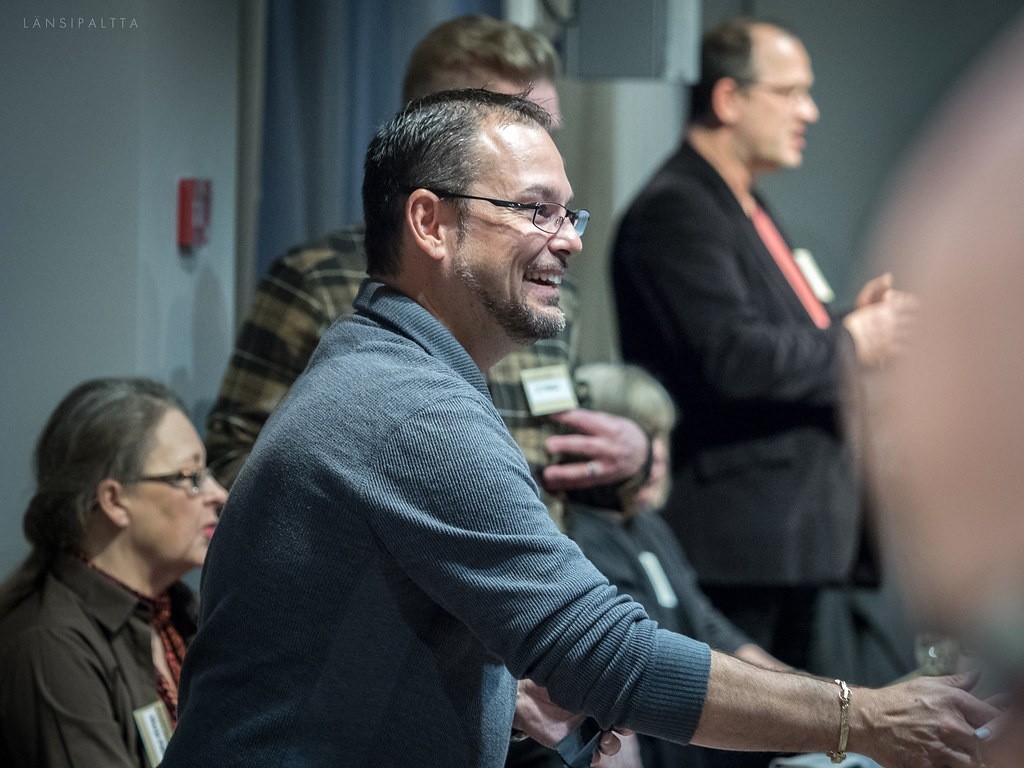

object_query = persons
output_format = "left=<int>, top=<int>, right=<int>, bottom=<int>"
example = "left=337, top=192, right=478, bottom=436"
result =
left=966, top=688, right=1022, bottom=766
left=199, top=18, right=651, bottom=766
left=0, top=377, right=234, bottom=768
left=610, top=14, right=924, bottom=765
left=565, top=358, right=824, bottom=767
left=157, top=84, right=1000, bottom=767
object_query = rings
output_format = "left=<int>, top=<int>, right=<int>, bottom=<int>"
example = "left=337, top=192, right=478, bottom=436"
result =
left=584, top=463, right=602, bottom=486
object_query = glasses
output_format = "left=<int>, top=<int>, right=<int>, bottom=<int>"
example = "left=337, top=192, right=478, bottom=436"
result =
left=89, top=467, right=213, bottom=516
left=400, top=184, right=590, bottom=239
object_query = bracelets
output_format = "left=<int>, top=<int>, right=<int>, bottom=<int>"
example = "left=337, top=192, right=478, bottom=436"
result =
left=822, top=676, right=853, bottom=766
